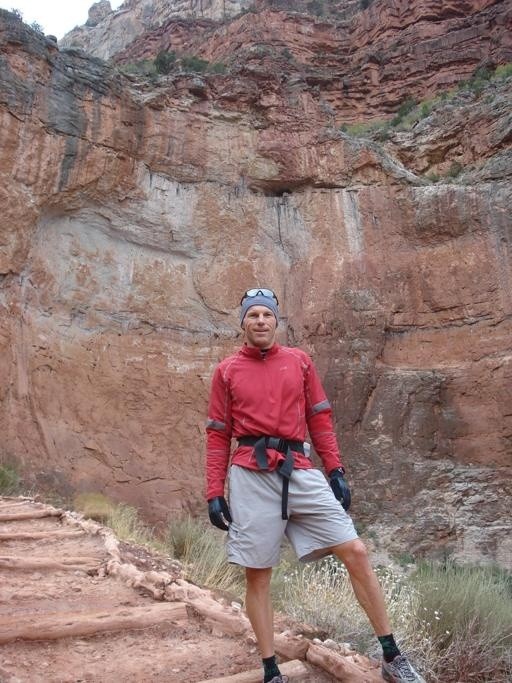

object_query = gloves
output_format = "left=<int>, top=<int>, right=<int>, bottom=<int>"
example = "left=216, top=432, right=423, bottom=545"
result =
left=329, top=467, right=351, bottom=511
left=208, top=496, right=232, bottom=530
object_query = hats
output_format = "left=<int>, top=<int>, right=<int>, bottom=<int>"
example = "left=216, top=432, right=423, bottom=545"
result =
left=239, top=294, right=280, bottom=332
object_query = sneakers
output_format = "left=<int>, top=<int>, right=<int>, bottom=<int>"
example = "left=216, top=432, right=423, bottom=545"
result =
left=263, top=672, right=285, bottom=683
left=382, top=654, right=427, bottom=683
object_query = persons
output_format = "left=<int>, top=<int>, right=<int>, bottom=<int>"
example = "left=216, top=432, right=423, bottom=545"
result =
left=204, top=284, right=427, bottom=683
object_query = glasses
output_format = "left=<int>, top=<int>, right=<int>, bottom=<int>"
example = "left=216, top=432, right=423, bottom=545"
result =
left=240, top=288, right=279, bottom=306
